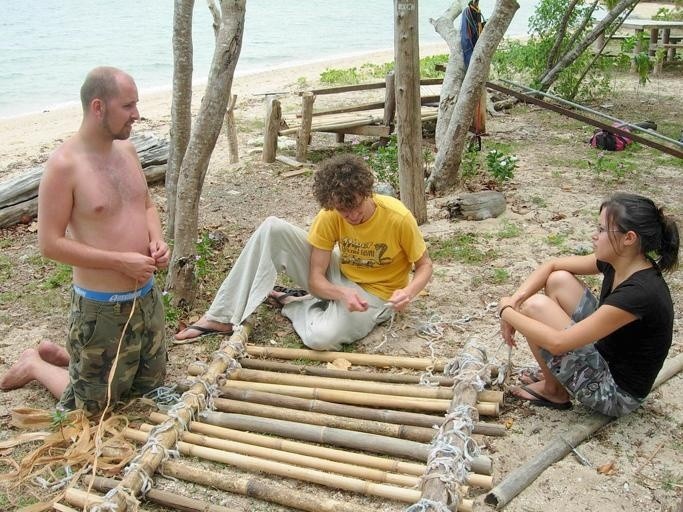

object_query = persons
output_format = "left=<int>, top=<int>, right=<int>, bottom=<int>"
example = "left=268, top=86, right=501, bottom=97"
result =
left=0, top=67, right=173, bottom=421
left=497, top=192, right=680, bottom=418
left=168, top=156, right=434, bottom=355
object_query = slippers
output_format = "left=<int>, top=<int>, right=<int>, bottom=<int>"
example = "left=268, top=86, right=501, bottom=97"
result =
left=267, top=285, right=307, bottom=308
left=517, top=367, right=546, bottom=383
left=171, top=321, right=234, bottom=345
left=510, top=383, right=573, bottom=412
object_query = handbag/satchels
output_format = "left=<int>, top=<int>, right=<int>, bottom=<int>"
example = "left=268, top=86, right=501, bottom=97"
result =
left=590, top=122, right=632, bottom=152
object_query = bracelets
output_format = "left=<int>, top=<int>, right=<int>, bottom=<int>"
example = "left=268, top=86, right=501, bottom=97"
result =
left=498, top=305, right=512, bottom=319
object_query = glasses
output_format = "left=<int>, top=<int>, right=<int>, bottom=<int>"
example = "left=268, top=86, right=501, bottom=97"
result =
left=595, top=225, right=620, bottom=235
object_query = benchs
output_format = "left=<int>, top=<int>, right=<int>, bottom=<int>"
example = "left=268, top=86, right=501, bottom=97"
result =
left=594, top=18, right=682, bottom=81
left=259, top=77, right=486, bottom=164
